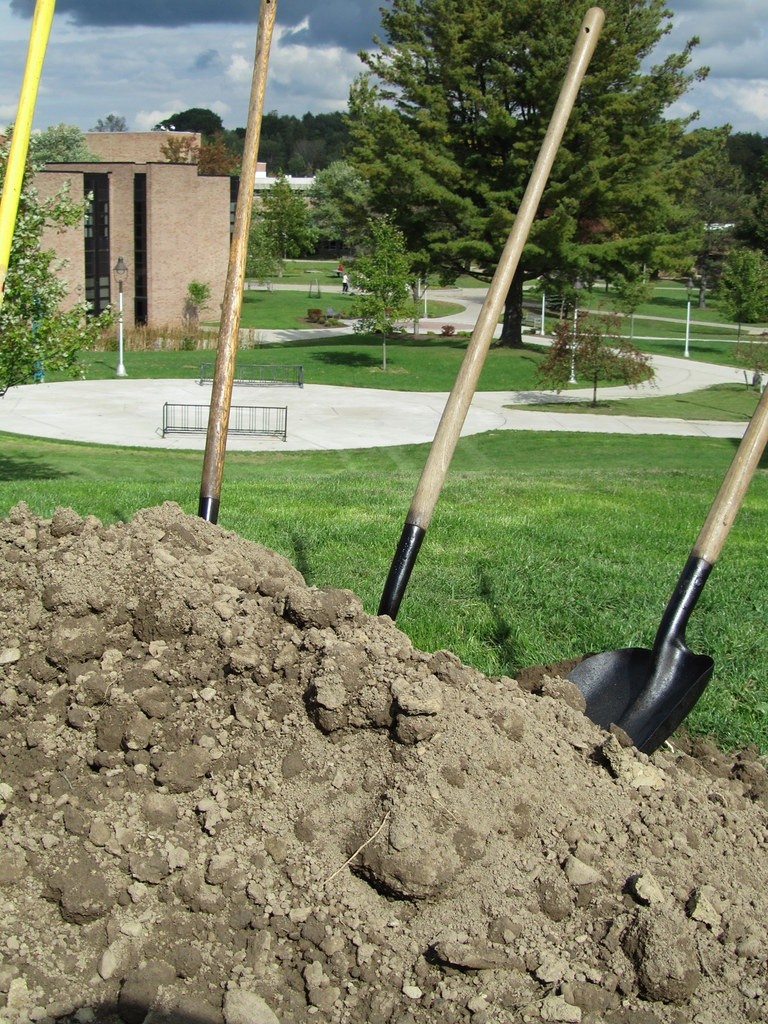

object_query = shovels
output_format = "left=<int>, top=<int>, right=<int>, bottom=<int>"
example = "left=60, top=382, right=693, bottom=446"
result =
left=565, top=382, right=767, bottom=751
left=201, top=2, right=278, bottom=525
left=380, top=3, right=609, bottom=626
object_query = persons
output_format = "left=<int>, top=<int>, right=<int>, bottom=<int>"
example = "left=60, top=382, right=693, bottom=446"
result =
left=342, top=273, right=348, bottom=292
left=337, top=265, right=342, bottom=276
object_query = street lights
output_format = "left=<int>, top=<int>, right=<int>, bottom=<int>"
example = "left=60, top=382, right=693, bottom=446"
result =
left=113, top=257, right=129, bottom=376
left=683, top=278, right=695, bottom=359
left=566, top=277, right=584, bottom=384
